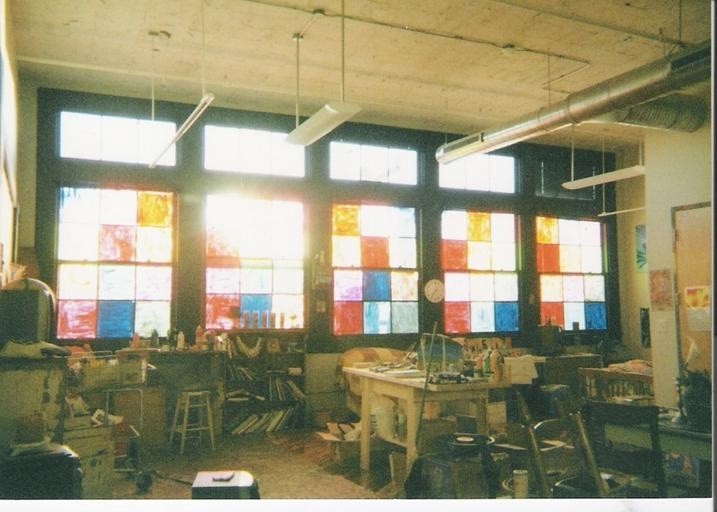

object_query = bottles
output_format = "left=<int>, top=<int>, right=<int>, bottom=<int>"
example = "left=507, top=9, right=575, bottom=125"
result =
left=176, top=331, right=184, bottom=349
left=472, top=338, right=503, bottom=384
left=195, top=325, right=204, bottom=344
left=150, top=329, right=159, bottom=347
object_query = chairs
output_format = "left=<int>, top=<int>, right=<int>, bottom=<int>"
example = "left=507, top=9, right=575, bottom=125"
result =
left=341, top=346, right=440, bottom=422
left=524, top=409, right=610, bottom=498
left=581, top=396, right=689, bottom=498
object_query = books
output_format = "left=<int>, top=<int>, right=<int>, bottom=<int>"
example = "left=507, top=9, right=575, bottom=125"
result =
left=224, top=335, right=307, bottom=436
left=584, top=375, right=647, bottom=400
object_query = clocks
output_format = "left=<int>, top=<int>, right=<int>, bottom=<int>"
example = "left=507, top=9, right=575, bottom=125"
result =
left=424, top=279, right=444, bottom=304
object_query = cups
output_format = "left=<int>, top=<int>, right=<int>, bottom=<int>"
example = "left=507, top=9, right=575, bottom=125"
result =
left=513, top=469, right=528, bottom=499
left=242, top=310, right=285, bottom=329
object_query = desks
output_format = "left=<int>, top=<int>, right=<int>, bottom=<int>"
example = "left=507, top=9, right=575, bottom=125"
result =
left=146, top=349, right=224, bottom=436
left=341, top=366, right=505, bottom=476
left=605, top=413, right=712, bottom=460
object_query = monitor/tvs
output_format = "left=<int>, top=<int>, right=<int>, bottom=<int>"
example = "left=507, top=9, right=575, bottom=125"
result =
left=0, top=288, right=55, bottom=349
left=535, top=325, right=564, bottom=355
left=190, top=468, right=262, bottom=499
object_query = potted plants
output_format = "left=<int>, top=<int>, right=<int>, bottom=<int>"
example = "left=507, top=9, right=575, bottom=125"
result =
left=676, top=367, right=712, bottom=426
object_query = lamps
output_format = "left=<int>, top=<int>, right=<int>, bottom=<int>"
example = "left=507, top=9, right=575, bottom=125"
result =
left=285, top=0, right=362, bottom=147
left=148, top=0, right=215, bottom=169
left=562, top=123, right=646, bottom=190
left=597, top=136, right=646, bottom=218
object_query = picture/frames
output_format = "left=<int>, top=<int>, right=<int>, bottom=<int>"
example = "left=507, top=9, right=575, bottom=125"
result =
left=643, top=442, right=704, bottom=492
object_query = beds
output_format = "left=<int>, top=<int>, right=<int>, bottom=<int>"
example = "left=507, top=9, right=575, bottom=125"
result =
left=577, top=360, right=653, bottom=401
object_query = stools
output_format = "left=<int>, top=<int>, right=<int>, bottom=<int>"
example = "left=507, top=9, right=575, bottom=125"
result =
left=168, top=388, right=216, bottom=455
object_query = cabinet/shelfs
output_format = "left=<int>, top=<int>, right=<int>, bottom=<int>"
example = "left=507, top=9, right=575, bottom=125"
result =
left=523, top=352, right=602, bottom=419
left=224, top=328, right=307, bottom=436
